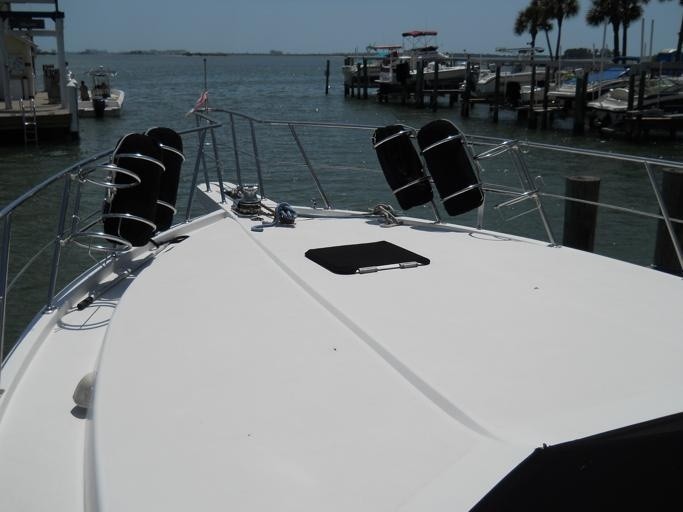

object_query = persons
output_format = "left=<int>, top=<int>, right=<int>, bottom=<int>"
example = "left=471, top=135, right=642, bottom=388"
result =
left=80, top=80, right=90, bottom=101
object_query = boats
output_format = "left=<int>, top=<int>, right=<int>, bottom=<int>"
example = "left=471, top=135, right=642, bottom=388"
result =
left=77, top=65, right=126, bottom=118
